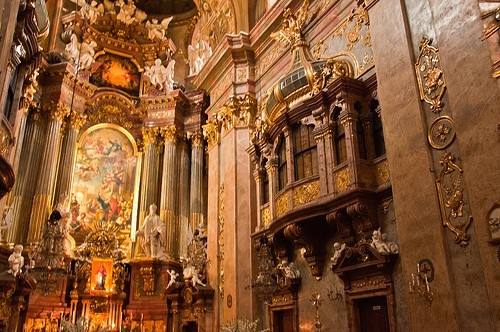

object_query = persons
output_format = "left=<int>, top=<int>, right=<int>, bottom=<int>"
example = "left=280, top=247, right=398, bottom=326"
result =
left=136, top=203, right=166, bottom=257
left=8, top=244, right=25, bottom=277
left=79, top=1, right=100, bottom=24
left=144, top=58, right=175, bottom=89
left=329, top=241, right=346, bottom=267
left=166, top=269, right=179, bottom=287
left=190, top=269, right=206, bottom=287
left=118, top=0, right=137, bottom=24
left=371, top=226, right=391, bottom=253
left=65, top=34, right=97, bottom=68
left=95, top=262, right=107, bottom=291
left=146, top=18, right=166, bottom=39
left=190, top=34, right=211, bottom=60
left=281, top=260, right=300, bottom=278
